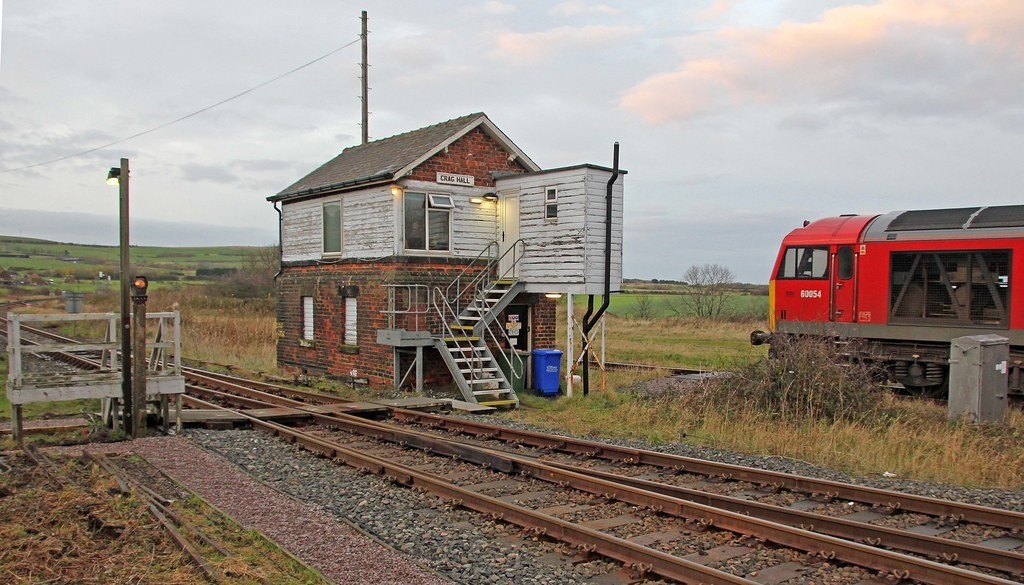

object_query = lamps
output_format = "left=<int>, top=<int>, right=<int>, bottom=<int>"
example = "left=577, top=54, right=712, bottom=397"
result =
left=391, top=185, right=403, bottom=194
left=484, top=192, right=497, bottom=200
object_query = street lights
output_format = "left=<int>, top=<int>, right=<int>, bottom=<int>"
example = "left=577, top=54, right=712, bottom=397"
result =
left=106, top=158, right=133, bottom=438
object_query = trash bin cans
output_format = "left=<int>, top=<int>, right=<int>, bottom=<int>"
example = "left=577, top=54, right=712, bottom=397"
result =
left=532, top=349, right=564, bottom=396
left=495, top=349, right=530, bottom=394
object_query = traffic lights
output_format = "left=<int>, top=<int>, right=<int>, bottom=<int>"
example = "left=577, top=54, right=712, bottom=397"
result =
left=130, top=276, right=148, bottom=295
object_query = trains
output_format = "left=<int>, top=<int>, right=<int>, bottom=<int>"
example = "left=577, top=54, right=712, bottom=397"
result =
left=749, top=205, right=1024, bottom=410
